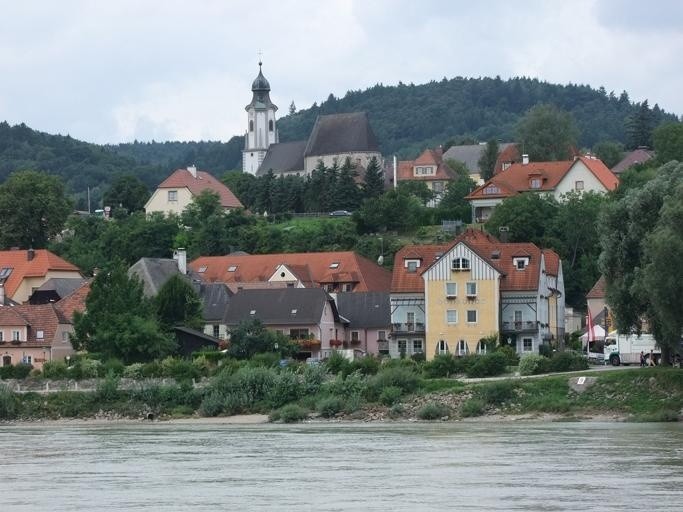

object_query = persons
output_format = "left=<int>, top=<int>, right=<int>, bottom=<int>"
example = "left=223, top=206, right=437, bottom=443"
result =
left=647, top=348, right=656, bottom=367
left=639, top=350, right=646, bottom=367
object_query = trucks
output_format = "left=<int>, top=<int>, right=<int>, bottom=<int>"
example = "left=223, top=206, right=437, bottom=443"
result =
left=604, top=326, right=664, bottom=366
